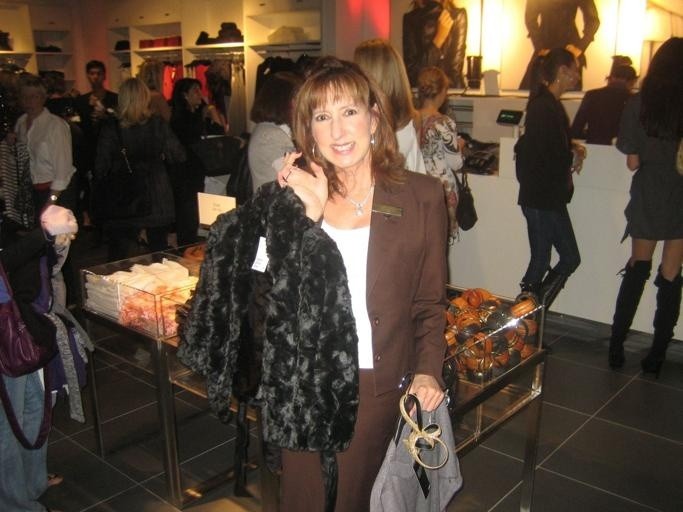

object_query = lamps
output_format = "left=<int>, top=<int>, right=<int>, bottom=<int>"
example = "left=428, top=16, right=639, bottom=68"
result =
left=640, top=9, right=672, bottom=63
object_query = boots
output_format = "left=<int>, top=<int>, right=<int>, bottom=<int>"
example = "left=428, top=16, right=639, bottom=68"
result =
left=520, top=259, right=575, bottom=355
left=641, top=263, right=682, bottom=379
left=608, top=259, right=652, bottom=377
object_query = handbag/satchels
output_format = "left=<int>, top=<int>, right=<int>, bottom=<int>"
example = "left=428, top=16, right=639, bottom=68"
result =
left=89, top=172, right=150, bottom=221
left=1, top=253, right=69, bottom=379
left=455, top=185, right=477, bottom=232
left=368, top=392, right=464, bottom=512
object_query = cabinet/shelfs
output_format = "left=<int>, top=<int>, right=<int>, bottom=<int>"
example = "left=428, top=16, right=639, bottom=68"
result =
left=79, top=241, right=550, bottom=512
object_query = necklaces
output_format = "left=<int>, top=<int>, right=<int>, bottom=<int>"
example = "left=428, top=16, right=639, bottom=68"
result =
left=344, top=179, right=374, bottom=217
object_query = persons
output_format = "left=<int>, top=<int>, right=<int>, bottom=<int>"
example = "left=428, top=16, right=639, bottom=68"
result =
left=571, top=55, right=639, bottom=145
left=607, top=36, right=683, bottom=380
left=402, top=0, right=468, bottom=88
left=514, top=49, right=587, bottom=352
left=519, top=0, right=600, bottom=94
left=413, top=66, right=465, bottom=283
left=276, top=57, right=450, bottom=511
left=1, top=61, right=232, bottom=512
left=354, top=38, right=426, bottom=174
left=248, top=72, right=305, bottom=194
